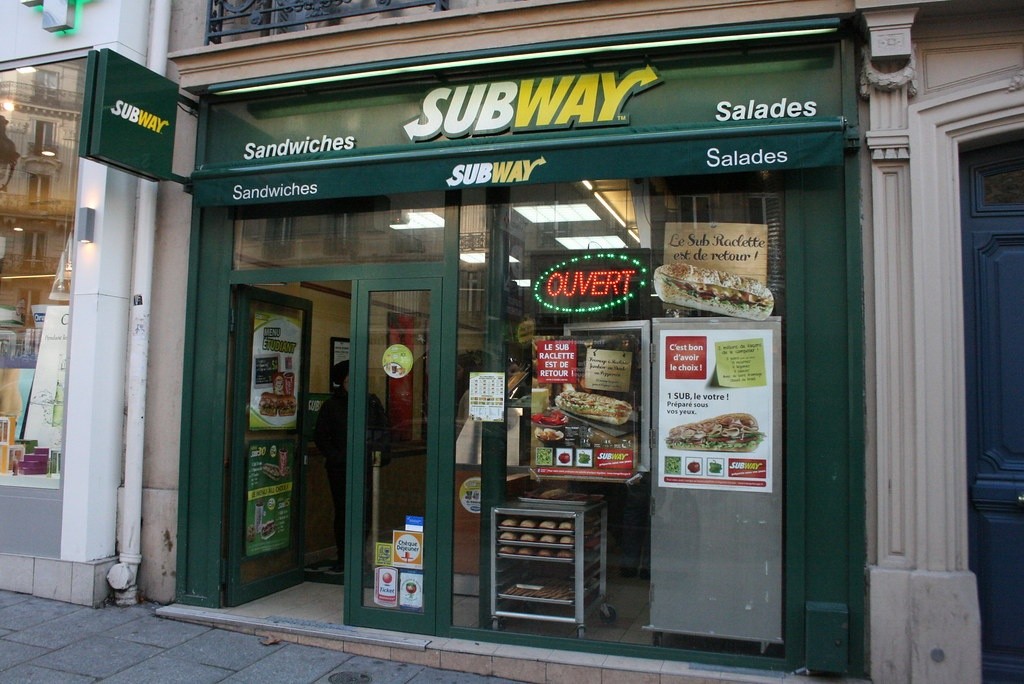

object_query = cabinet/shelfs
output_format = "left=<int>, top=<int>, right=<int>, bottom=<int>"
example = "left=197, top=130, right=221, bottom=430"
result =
left=488, top=500, right=608, bottom=628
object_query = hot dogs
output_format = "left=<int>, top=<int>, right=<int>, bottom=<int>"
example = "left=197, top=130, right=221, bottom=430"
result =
left=653, top=263, right=774, bottom=321
left=665, top=413, right=767, bottom=453
left=555, top=391, right=633, bottom=425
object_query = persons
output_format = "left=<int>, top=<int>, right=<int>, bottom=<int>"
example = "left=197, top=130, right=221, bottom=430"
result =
left=314, top=359, right=392, bottom=574
left=508, top=359, right=519, bottom=380
left=456, top=358, right=481, bottom=441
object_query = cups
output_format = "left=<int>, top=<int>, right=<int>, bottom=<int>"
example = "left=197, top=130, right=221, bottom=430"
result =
left=0, top=328, right=42, bottom=361
left=271, top=371, right=294, bottom=395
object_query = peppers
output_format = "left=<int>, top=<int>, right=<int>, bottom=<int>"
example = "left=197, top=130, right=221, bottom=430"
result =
left=579, top=450, right=590, bottom=463
left=710, top=460, right=721, bottom=474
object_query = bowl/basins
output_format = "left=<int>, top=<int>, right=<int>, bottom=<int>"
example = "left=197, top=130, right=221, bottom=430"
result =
left=535, top=430, right=565, bottom=444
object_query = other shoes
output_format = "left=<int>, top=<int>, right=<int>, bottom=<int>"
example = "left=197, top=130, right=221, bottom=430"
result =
left=622, top=566, right=649, bottom=577
left=323, top=561, right=344, bottom=574
left=362, top=560, right=372, bottom=574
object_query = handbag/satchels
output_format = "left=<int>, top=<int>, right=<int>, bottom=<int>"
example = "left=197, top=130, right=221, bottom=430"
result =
left=369, top=394, right=392, bottom=465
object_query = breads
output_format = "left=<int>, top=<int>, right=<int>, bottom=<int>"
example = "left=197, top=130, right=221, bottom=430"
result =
left=499, top=515, right=599, bottom=557
left=540, top=489, right=567, bottom=500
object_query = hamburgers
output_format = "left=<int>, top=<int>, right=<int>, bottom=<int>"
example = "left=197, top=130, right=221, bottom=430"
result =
left=258, top=392, right=296, bottom=417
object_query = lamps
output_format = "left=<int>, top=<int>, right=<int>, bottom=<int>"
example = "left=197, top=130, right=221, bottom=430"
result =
left=42, top=121, right=56, bottom=156
left=77, top=206, right=95, bottom=243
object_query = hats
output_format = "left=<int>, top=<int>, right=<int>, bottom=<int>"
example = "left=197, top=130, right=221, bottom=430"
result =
left=333, top=359, right=349, bottom=385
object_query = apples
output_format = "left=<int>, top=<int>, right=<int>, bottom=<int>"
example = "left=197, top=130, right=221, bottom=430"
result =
left=687, top=460, right=700, bottom=472
left=558, top=452, right=570, bottom=463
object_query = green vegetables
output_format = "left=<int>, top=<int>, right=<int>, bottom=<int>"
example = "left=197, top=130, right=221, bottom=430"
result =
left=537, top=448, right=551, bottom=464
left=665, top=456, right=682, bottom=476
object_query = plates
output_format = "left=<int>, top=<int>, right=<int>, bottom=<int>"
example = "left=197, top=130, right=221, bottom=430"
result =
left=536, top=423, right=563, bottom=427
left=251, top=397, right=297, bottom=426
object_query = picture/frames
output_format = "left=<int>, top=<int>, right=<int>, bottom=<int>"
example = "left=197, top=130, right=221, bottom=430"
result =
left=328, top=336, right=350, bottom=392
